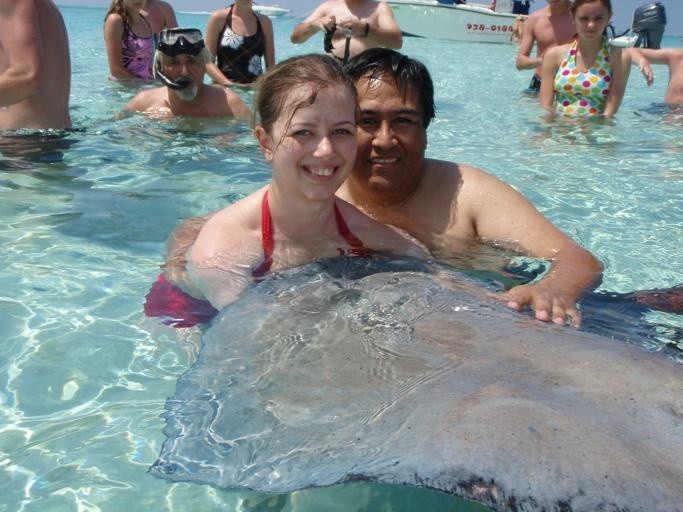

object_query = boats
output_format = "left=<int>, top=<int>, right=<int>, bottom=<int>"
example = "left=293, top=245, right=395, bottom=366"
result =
left=388, top=1, right=665, bottom=48
left=250, top=5, right=291, bottom=21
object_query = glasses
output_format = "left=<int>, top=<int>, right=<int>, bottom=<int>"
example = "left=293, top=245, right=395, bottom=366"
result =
left=158, top=27, right=205, bottom=56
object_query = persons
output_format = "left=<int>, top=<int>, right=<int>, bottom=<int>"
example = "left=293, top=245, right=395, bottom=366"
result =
left=0, top=0, right=72, bottom=173
left=102, top=1, right=155, bottom=84
left=200, top=0, right=275, bottom=92
left=138, top=0, right=180, bottom=31
left=165, top=45, right=604, bottom=331
left=512, top=16, right=525, bottom=41
left=79, top=27, right=257, bottom=146
left=437, top=0, right=496, bottom=11
left=534, top=2, right=630, bottom=148
left=514, top=0, right=583, bottom=96
left=138, top=50, right=502, bottom=329
left=288, top=0, right=403, bottom=67
left=625, top=46, right=683, bottom=105
